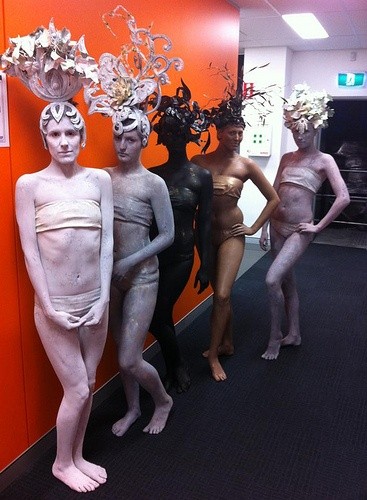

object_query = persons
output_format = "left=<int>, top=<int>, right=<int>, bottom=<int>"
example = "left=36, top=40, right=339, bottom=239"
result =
left=190, top=128, right=280, bottom=380
left=15, top=101, right=114, bottom=492
left=101, top=106, right=174, bottom=434
left=147, top=115, right=214, bottom=393
left=259, top=124, right=350, bottom=361
